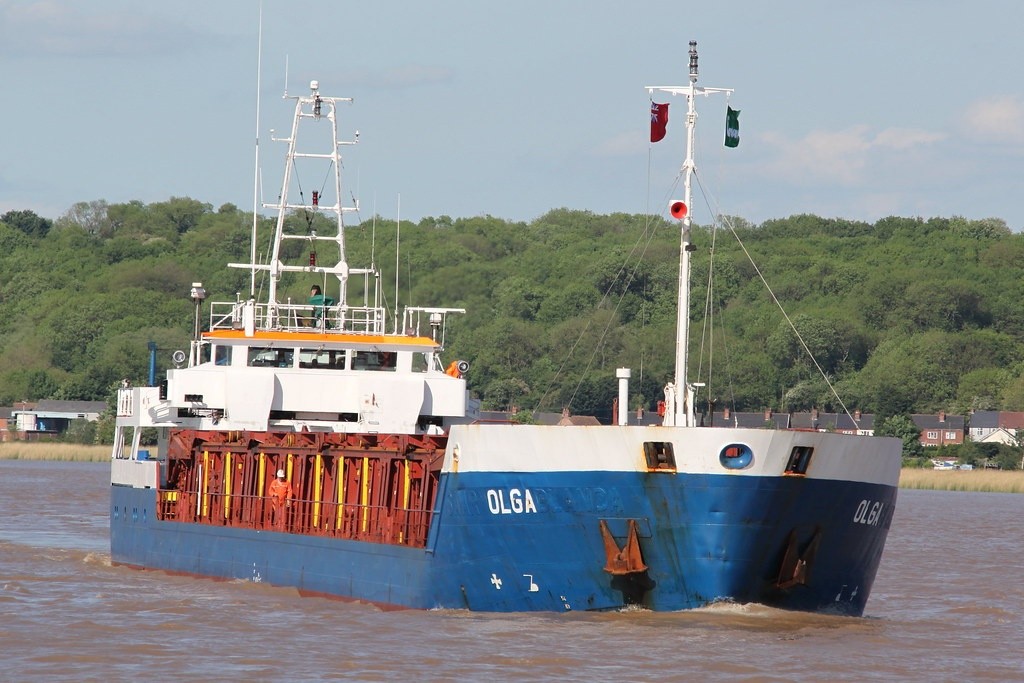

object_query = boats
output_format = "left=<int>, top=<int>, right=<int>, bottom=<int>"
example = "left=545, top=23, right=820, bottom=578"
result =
left=107, top=38, right=907, bottom=618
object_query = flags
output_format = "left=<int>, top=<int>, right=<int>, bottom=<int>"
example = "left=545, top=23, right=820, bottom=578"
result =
left=724, top=105, right=741, bottom=148
left=650, top=101, right=670, bottom=142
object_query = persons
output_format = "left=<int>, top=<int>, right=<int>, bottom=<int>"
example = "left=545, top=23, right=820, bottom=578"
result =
left=311, top=283, right=329, bottom=327
